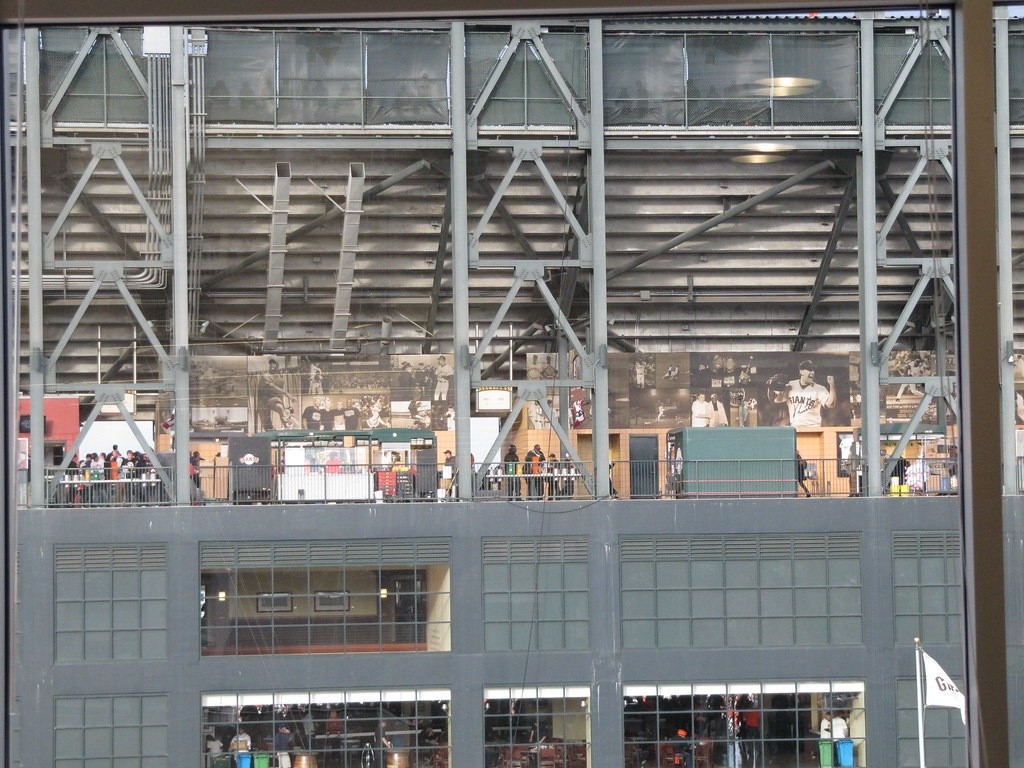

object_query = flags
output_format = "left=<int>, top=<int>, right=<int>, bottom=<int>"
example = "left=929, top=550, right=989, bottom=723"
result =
left=162, top=408, right=175, bottom=433
left=923, top=651, right=965, bottom=725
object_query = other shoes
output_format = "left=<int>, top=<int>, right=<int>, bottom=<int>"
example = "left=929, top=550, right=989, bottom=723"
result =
left=507, top=498, right=512, bottom=500
left=806, top=494, right=811, bottom=496
left=517, top=497, right=523, bottom=500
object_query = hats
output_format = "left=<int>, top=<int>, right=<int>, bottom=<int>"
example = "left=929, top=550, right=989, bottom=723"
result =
left=798, top=359, right=816, bottom=370
left=444, top=450, right=450, bottom=453
left=402, top=360, right=410, bottom=367
left=548, top=452, right=554, bottom=456
left=278, top=723, right=287, bottom=728
left=270, top=358, right=278, bottom=365
left=508, top=444, right=516, bottom=450
left=438, top=356, right=446, bottom=360
left=534, top=445, right=541, bottom=449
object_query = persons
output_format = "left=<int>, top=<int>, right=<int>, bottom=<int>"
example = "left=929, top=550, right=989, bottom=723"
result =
left=818, top=710, right=848, bottom=742
left=205, top=711, right=443, bottom=768
left=257, top=358, right=297, bottom=431
left=634, top=358, right=684, bottom=422
left=212, top=452, right=286, bottom=505
left=306, top=452, right=342, bottom=474
left=690, top=355, right=751, bottom=428
left=795, top=450, right=812, bottom=498
left=529, top=719, right=546, bottom=766
left=400, top=355, right=454, bottom=431
left=888, top=455, right=910, bottom=494
left=528, top=354, right=588, bottom=430
left=893, top=352, right=931, bottom=402
left=382, top=451, right=391, bottom=464
left=625, top=704, right=760, bottom=768
left=52, top=444, right=169, bottom=508
left=189, top=451, right=207, bottom=506
left=299, top=356, right=392, bottom=430
left=504, top=444, right=620, bottom=502
left=767, top=361, right=837, bottom=427
left=391, top=455, right=408, bottom=492
left=443, top=450, right=459, bottom=501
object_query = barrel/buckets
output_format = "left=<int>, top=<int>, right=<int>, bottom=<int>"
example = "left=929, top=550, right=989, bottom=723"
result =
left=386, top=752, right=410, bottom=768
left=293, top=755, right=318, bottom=768
left=889, top=485, right=910, bottom=497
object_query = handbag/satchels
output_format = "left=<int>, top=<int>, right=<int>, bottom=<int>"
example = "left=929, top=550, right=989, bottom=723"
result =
left=800, top=459, right=806, bottom=467
